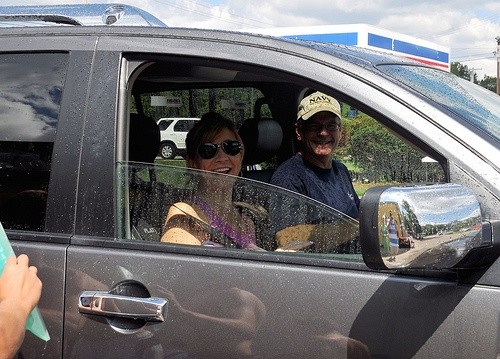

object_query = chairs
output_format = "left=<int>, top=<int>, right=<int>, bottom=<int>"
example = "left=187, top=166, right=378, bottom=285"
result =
left=130, top=112, right=179, bottom=241
left=236, top=116, right=283, bottom=204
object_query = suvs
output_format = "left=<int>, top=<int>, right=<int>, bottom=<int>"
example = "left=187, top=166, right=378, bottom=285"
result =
left=0, top=4, right=500, bottom=358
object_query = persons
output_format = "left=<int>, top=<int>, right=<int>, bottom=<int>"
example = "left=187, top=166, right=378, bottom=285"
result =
left=380, top=214, right=400, bottom=261
left=0, top=255, right=42, bottom=359
left=267, top=91, right=362, bottom=254
left=161, top=112, right=257, bottom=249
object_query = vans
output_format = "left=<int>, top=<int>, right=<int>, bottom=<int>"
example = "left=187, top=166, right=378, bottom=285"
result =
left=155, top=117, right=202, bottom=159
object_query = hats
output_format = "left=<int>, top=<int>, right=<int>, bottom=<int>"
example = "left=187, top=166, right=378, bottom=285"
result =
left=296, top=90, right=342, bottom=121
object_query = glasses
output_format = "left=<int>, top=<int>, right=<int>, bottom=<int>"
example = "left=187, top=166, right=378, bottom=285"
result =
left=191, top=140, right=243, bottom=159
left=300, top=122, right=341, bottom=131
